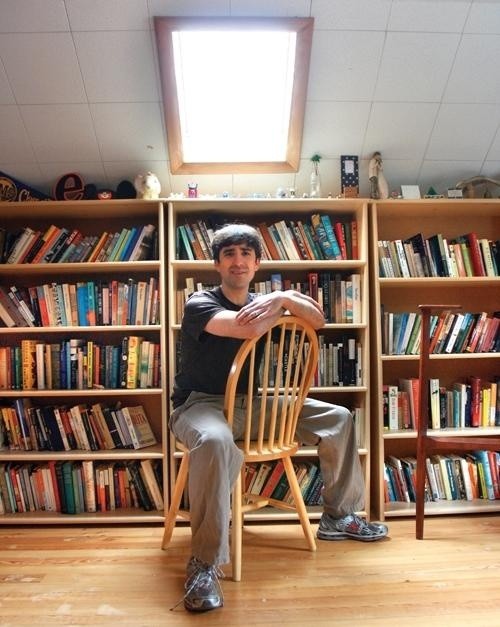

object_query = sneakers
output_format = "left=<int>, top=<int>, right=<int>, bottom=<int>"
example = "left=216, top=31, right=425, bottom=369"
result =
left=182, top=554, right=224, bottom=611
left=315, top=511, right=389, bottom=542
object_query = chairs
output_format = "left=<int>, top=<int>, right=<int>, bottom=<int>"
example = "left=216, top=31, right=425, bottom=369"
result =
left=155, top=311, right=322, bottom=583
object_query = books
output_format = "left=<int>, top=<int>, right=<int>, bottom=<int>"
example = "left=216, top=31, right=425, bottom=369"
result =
left=353, top=404, right=363, bottom=449
left=2, top=397, right=157, bottom=452
left=258, top=332, right=362, bottom=387
left=2, top=276, right=161, bottom=328
left=2, top=335, right=162, bottom=390
left=179, top=459, right=325, bottom=505
left=179, top=214, right=358, bottom=262
left=377, top=231, right=500, bottom=505
left=3, top=460, right=162, bottom=511
left=177, top=270, right=361, bottom=323
left=2, top=223, right=159, bottom=261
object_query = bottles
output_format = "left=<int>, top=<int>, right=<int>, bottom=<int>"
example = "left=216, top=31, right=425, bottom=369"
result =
left=310, top=160, right=322, bottom=198
left=188, top=183, right=198, bottom=198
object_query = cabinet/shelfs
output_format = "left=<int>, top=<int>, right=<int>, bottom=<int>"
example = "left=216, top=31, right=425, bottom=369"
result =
left=1, top=195, right=174, bottom=526
left=165, top=198, right=373, bottom=526
left=367, top=196, right=500, bottom=529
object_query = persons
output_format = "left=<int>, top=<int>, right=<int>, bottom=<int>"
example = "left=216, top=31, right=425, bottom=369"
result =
left=167, top=223, right=388, bottom=613
left=367, top=152, right=389, bottom=199
